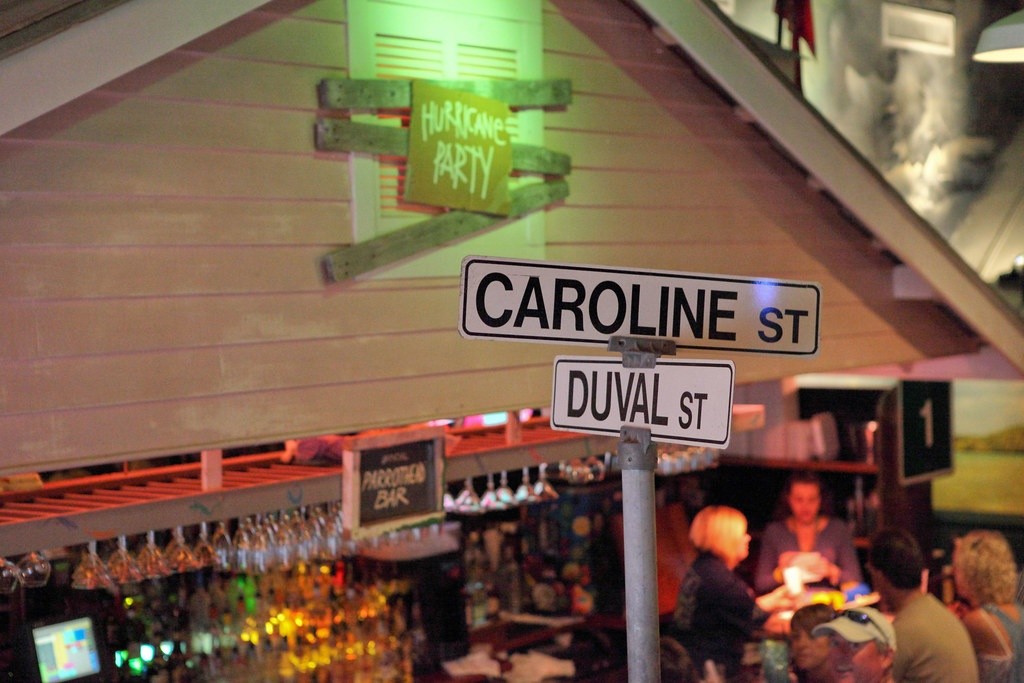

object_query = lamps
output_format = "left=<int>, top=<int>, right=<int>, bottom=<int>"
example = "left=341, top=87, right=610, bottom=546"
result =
left=972, top=8, right=1024, bottom=65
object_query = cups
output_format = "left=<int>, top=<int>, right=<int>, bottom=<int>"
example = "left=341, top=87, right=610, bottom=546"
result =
left=18, top=552, right=51, bottom=589
left=760, top=637, right=790, bottom=682
left=0, top=557, right=18, bottom=594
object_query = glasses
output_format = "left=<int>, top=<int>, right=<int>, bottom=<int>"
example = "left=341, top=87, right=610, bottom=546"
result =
left=831, top=610, right=891, bottom=640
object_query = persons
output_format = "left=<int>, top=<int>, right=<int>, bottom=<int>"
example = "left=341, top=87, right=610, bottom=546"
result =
left=675, top=475, right=1024, bottom=683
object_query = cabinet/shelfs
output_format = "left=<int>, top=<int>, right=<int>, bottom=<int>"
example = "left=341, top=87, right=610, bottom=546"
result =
left=739, top=391, right=933, bottom=498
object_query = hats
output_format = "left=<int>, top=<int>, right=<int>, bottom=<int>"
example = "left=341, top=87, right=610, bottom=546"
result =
left=812, top=606, right=897, bottom=653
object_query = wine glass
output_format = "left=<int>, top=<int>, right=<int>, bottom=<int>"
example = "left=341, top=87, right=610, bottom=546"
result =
left=444, top=464, right=560, bottom=515
left=71, top=506, right=342, bottom=591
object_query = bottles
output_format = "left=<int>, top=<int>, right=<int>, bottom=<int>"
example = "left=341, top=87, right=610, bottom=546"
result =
left=463, top=524, right=528, bottom=626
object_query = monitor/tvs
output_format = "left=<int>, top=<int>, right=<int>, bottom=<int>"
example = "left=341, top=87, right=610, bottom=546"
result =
left=23, top=610, right=114, bottom=683
